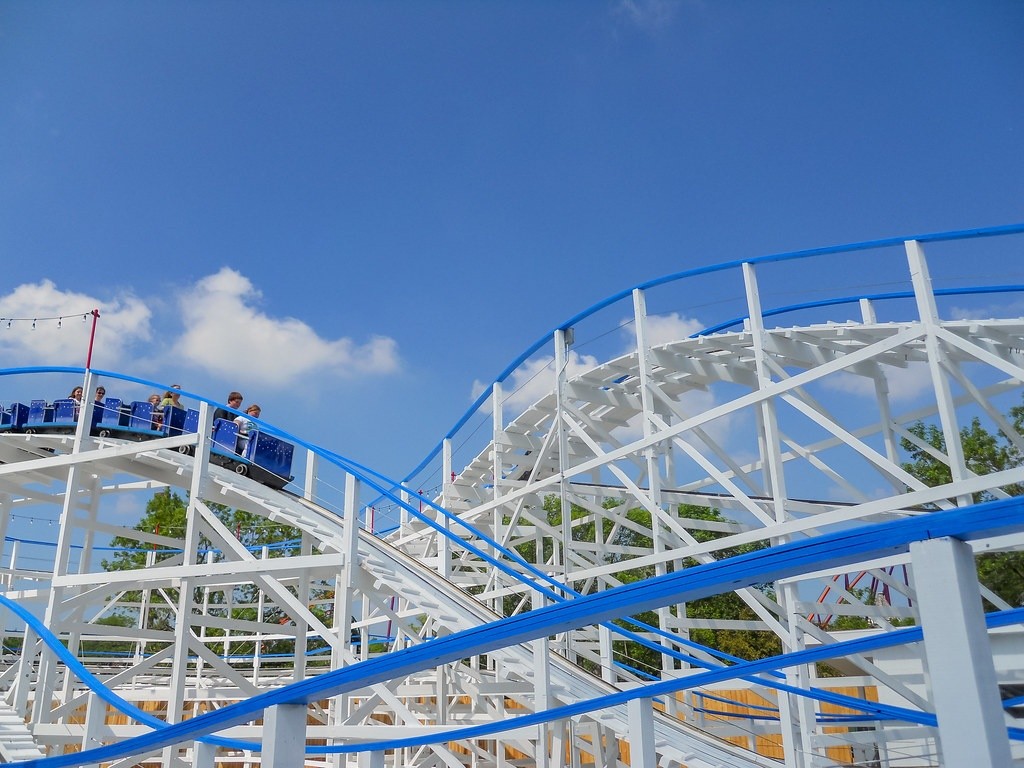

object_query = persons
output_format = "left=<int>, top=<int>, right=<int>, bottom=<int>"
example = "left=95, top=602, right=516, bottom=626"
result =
left=148, top=384, right=184, bottom=431
left=213, top=392, right=262, bottom=457
left=95, top=386, right=105, bottom=402
left=68, top=386, right=83, bottom=416
